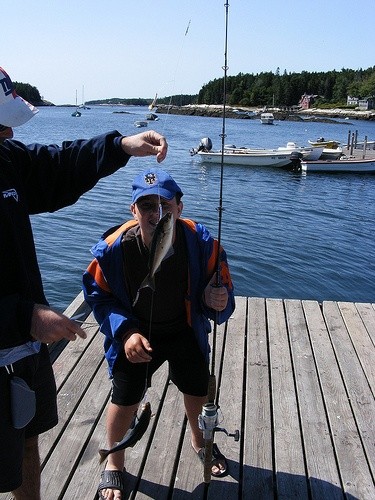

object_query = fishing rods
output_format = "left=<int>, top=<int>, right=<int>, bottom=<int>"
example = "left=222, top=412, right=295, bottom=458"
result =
left=199, top=0, right=243, bottom=500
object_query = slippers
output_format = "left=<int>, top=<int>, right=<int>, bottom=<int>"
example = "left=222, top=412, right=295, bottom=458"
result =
left=98, top=460, right=126, bottom=500
left=190, top=437, right=231, bottom=477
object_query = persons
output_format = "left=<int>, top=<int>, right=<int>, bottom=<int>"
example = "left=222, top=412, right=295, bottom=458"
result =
left=81, top=169, right=236, bottom=500
left=0, top=68, right=169, bottom=500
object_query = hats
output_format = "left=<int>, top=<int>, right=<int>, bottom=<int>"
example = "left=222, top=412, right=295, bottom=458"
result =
left=129, top=169, right=184, bottom=206
left=0, top=68, right=39, bottom=129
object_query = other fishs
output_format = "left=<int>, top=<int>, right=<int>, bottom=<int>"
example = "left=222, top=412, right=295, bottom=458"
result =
left=137, top=211, right=174, bottom=293
left=98, top=402, right=152, bottom=465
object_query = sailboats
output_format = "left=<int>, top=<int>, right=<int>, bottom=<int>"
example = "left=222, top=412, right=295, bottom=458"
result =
left=71, top=89, right=81, bottom=117
left=145, top=93, right=160, bottom=121
left=80, top=85, right=85, bottom=109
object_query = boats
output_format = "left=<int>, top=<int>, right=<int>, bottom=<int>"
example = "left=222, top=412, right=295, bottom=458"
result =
left=112, top=110, right=135, bottom=114
left=84, top=106, right=91, bottom=110
left=260, top=112, right=274, bottom=124
left=308, top=138, right=344, bottom=159
left=133, top=120, right=148, bottom=127
left=189, top=137, right=326, bottom=169
